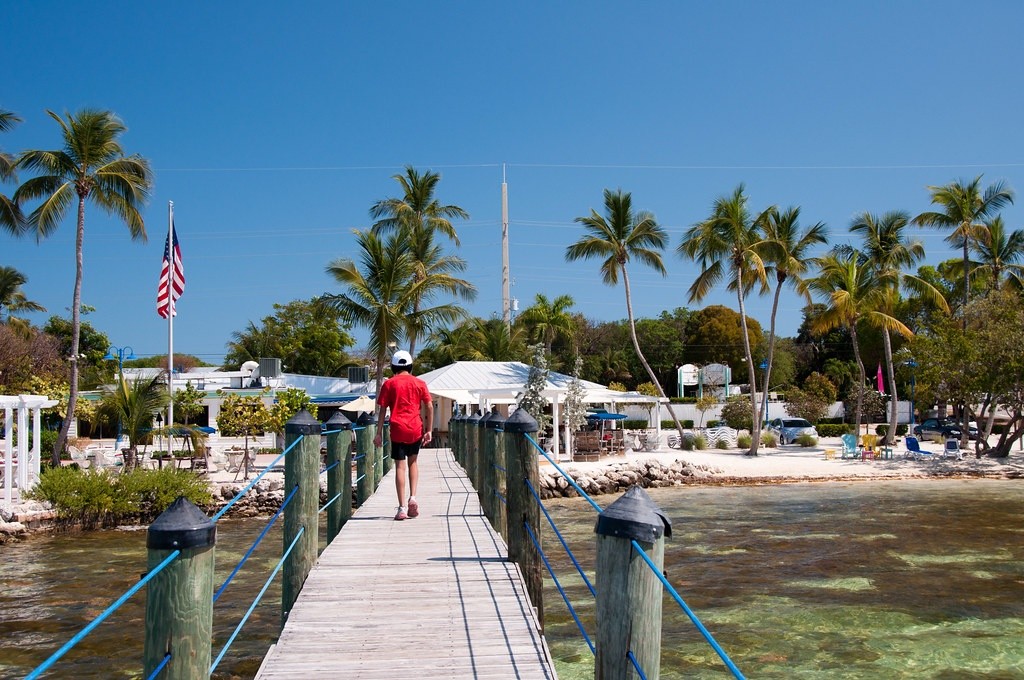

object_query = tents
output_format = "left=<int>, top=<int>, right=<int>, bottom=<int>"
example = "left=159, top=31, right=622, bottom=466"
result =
left=584, top=413, right=628, bottom=452
left=338, top=396, right=376, bottom=413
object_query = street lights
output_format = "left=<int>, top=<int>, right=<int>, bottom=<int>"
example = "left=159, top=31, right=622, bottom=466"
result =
left=905, top=359, right=917, bottom=436
left=761, top=358, right=774, bottom=428
left=106, top=347, right=137, bottom=448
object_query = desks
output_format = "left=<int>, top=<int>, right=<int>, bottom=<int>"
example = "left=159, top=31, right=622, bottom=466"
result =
left=163, top=457, right=195, bottom=469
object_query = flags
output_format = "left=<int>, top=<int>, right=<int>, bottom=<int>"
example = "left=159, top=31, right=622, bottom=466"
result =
left=157, top=211, right=185, bottom=319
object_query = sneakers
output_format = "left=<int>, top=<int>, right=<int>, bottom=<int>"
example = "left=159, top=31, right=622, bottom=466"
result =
left=394, top=506, right=406, bottom=520
left=407, top=498, right=418, bottom=517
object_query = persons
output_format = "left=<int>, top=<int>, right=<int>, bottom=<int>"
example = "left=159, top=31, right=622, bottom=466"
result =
left=373, top=351, right=433, bottom=520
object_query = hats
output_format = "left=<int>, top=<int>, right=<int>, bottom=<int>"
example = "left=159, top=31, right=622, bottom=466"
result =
left=392, top=350, right=412, bottom=366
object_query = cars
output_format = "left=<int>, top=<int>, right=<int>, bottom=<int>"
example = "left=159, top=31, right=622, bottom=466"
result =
left=915, top=417, right=983, bottom=442
left=768, top=419, right=819, bottom=445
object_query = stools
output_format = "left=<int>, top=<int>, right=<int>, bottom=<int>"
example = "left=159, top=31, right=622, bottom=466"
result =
left=862, top=451, right=873, bottom=461
left=825, top=450, right=835, bottom=460
left=880, top=448, right=892, bottom=460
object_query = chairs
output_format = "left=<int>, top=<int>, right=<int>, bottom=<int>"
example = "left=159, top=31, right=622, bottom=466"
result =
left=575, top=429, right=666, bottom=461
left=904, top=437, right=935, bottom=463
left=943, top=438, right=972, bottom=461
left=841, top=434, right=862, bottom=460
left=863, top=435, right=880, bottom=460
left=65, top=443, right=259, bottom=475
